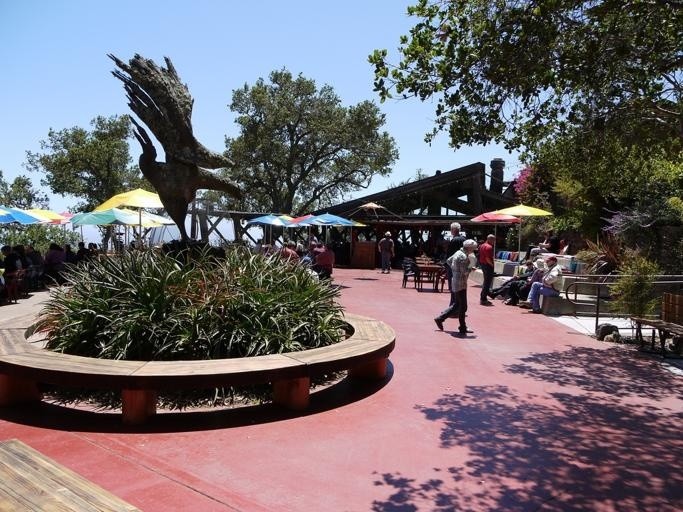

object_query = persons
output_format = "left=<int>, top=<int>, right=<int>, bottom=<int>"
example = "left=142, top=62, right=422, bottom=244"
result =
left=0, top=241, right=98, bottom=305
left=487, top=229, right=568, bottom=313
left=376, top=231, right=396, bottom=274
left=255, top=232, right=336, bottom=279
left=431, top=239, right=478, bottom=337
left=477, top=233, right=496, bottom=307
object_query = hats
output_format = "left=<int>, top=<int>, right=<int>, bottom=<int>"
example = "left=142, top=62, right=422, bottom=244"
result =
left=546, top=257, right=557, bottom=262
left=463, top=239, right=478, bottom=250
left=385, top=231, right=391, bottom=236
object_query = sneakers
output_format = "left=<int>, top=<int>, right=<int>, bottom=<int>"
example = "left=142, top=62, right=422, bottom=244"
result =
left=505, top=301, right=539, bottom=313
left=481, top=290, right=495, bottom=305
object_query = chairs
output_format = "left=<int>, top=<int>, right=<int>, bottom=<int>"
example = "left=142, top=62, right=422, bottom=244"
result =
left=401, top=256, right=453, bottom=292
left=0, top=255, right=84, bottom=304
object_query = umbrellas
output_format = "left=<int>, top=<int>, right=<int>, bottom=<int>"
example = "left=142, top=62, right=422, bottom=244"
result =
left=469, top=207, right=520, bottom=259
left=92, top=188, right=165, bottom=246
left=0, top=206, right=175, bottom=250
left=246, top=213, right=366, bottom=240
left=495, top=203, right=553, bottom=250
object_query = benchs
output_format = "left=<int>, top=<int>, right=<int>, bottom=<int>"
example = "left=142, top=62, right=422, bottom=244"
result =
left=493, top=245, right=577, bottom=277
left=630, top=292, right=683, bottom=360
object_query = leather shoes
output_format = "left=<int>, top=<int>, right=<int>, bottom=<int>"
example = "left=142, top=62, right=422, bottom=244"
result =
left=435, top=319, right=443, bottom=330
left=460, top=329, right=473, bottom=333
